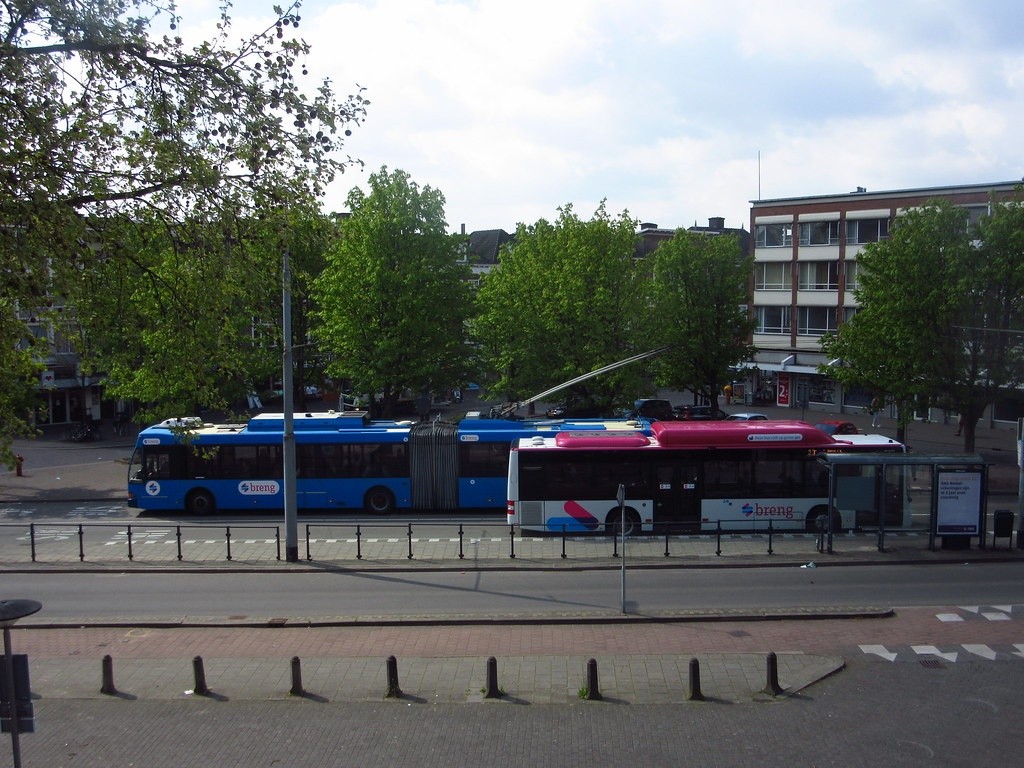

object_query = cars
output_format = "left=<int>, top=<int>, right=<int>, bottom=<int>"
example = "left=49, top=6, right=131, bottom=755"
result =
left=545, top=399, right=769, bottom=443
left=814, top=419, right=858, bottom=436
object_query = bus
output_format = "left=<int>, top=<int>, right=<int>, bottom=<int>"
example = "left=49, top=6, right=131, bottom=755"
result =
left=127, top=340, right=672, bottom=516
left=507, top=420, right=917, bottom=536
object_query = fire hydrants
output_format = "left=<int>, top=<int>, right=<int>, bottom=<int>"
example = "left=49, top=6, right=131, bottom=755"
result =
left=16, top=453, right=24, bottom=476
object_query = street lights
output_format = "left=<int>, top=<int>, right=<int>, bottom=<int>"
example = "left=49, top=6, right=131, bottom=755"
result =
left=1, top=599, right=43, bottom=768
